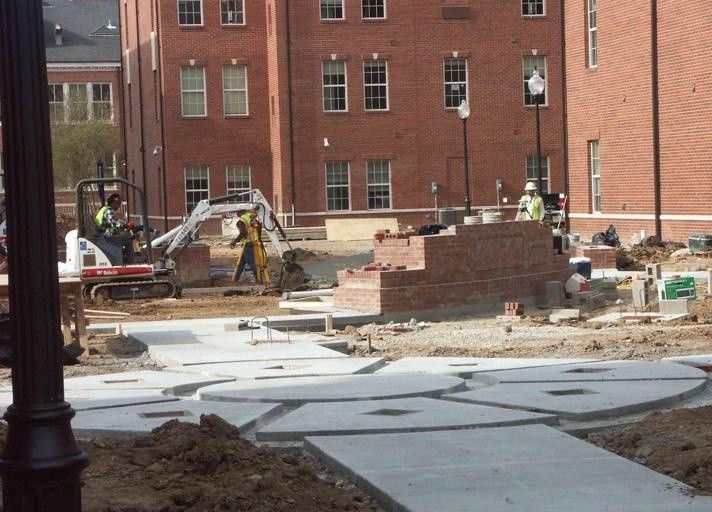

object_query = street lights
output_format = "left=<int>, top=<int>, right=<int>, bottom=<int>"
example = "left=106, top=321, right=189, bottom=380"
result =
left=457, top=99, right=475, bottom=214
left=527, top=64, right=546, bottom=203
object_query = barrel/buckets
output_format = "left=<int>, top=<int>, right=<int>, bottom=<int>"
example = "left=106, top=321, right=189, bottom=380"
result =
left=568, top=232, right=579, bottom=247
left=482, top=212, right=503, bottom=223
left=464, top=216, right=483, bottom=226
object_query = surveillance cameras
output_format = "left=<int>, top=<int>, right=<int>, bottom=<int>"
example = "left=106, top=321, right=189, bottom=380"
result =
left=153, top=151, right=158, bottom=156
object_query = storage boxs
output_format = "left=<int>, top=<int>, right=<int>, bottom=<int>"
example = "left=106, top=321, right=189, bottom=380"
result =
left=686, top=231, right=711, bottom=254
left=656, top=274, right=698, bottom=303
left=567, top=255, right=593, bottom=281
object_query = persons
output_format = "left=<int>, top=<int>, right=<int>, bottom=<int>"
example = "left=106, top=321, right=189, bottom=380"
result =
left=514, top=182, right=545, bottom=226
left=229, top=210, right=263, bottom=286
left=95, top=194, right=143, bottom=262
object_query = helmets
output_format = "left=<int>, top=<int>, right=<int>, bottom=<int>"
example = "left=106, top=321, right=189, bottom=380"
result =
left=524, top=181, right=537, bottom=192
left=107, top=193, right=122, bottom=204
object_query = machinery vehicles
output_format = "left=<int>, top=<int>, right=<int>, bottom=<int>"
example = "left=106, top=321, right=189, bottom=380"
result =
left=73, top=175, right=304, bottom=303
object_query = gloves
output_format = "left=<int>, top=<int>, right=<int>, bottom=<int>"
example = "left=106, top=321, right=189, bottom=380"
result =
left=126, top=221, right=135, bottom=230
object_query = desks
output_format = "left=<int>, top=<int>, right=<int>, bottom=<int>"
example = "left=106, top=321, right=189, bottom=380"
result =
left=0, top=273, right=97, bottom=359
